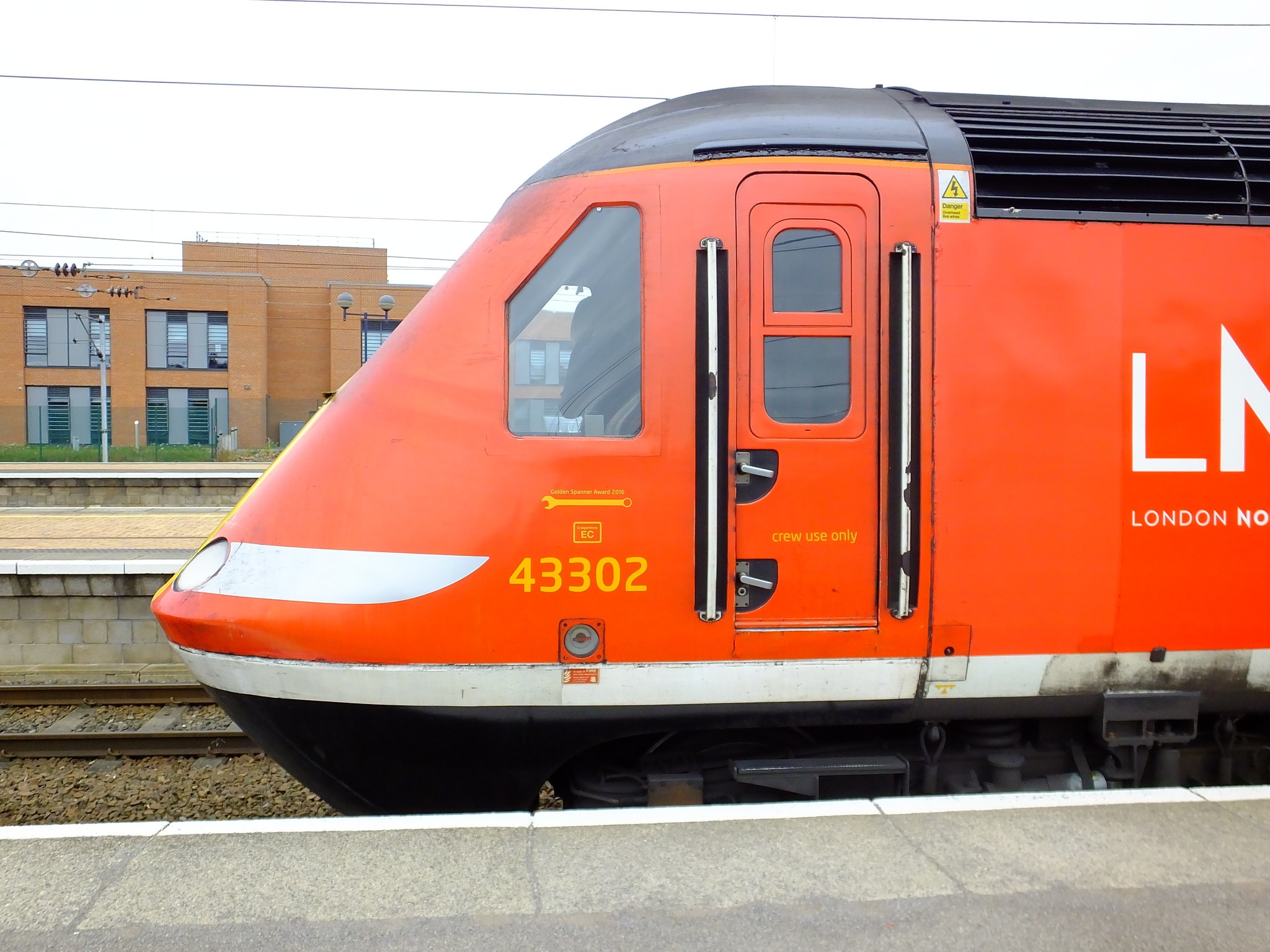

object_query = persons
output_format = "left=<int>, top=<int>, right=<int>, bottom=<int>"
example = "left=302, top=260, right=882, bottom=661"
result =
left=558, top=297, right=642, bottom=434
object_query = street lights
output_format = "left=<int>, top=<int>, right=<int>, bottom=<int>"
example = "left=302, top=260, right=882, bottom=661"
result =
left=338, top=293, right=396, bottom=363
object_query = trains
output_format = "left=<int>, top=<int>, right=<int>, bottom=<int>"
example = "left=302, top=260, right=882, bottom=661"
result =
left=163, top=82, right=1268, bottom=818
